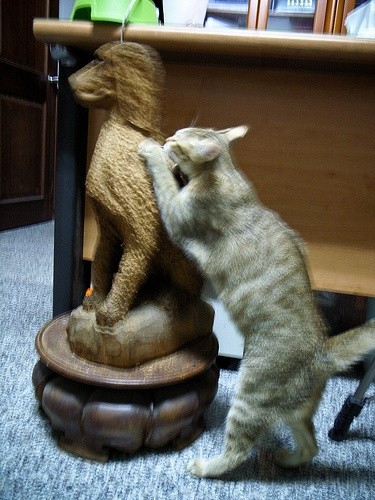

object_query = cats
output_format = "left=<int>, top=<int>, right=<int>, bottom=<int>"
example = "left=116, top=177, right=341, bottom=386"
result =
left=135, top=115, right=375, bottom=479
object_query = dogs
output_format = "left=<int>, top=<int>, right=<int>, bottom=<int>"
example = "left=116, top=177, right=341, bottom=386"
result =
left=67, top=41, right=204, bottom=327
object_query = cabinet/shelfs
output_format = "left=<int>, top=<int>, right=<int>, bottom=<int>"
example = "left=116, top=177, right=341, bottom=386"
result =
left=201, top=0, right=357, bottom=37
left=33, top=17, right=374, bottom=331
